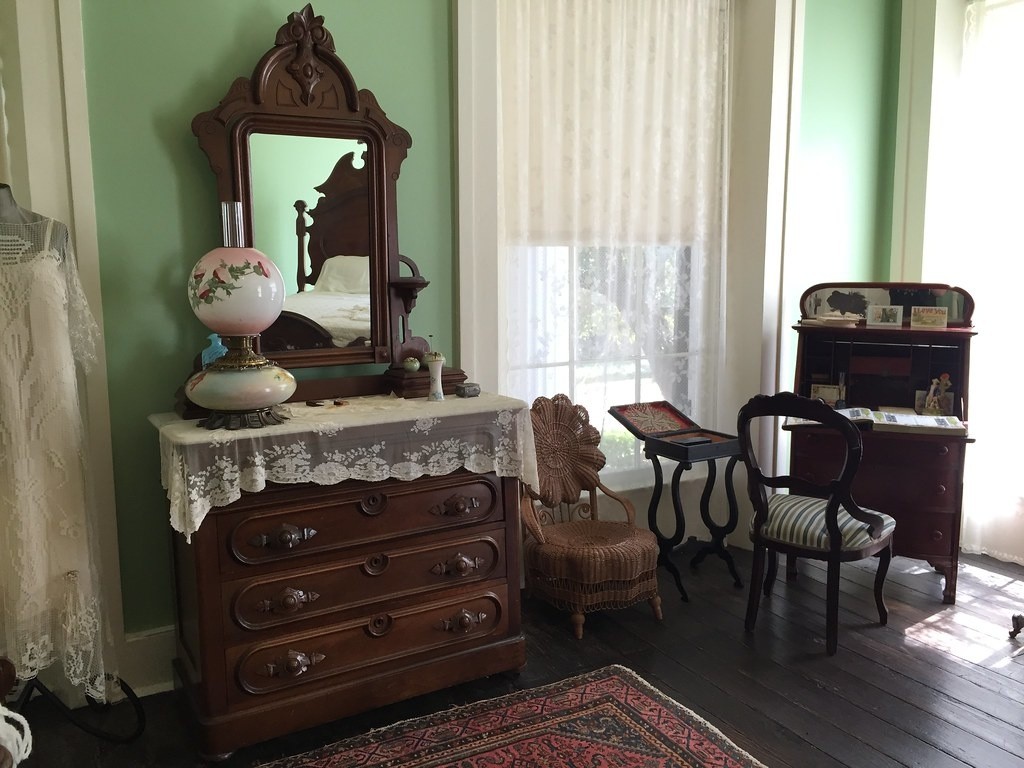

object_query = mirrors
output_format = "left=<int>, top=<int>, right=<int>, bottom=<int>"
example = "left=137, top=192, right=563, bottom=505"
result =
left=800, top=282, right=975, bottom=326
left=174, top=2, right=471, bottom=421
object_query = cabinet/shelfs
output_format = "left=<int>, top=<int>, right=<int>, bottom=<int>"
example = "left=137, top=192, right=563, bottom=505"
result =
left=782, top=321, right=977, bottom=603
left=145, top=368, right=528, bottom=760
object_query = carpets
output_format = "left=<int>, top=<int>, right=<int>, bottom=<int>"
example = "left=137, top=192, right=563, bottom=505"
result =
left=257, top=664, right=773, bottom=768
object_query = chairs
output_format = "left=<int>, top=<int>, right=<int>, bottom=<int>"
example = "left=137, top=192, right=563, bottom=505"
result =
left=736, top=392, right=897, bottom=656
left=521, top=393, right=663, bottom=638
